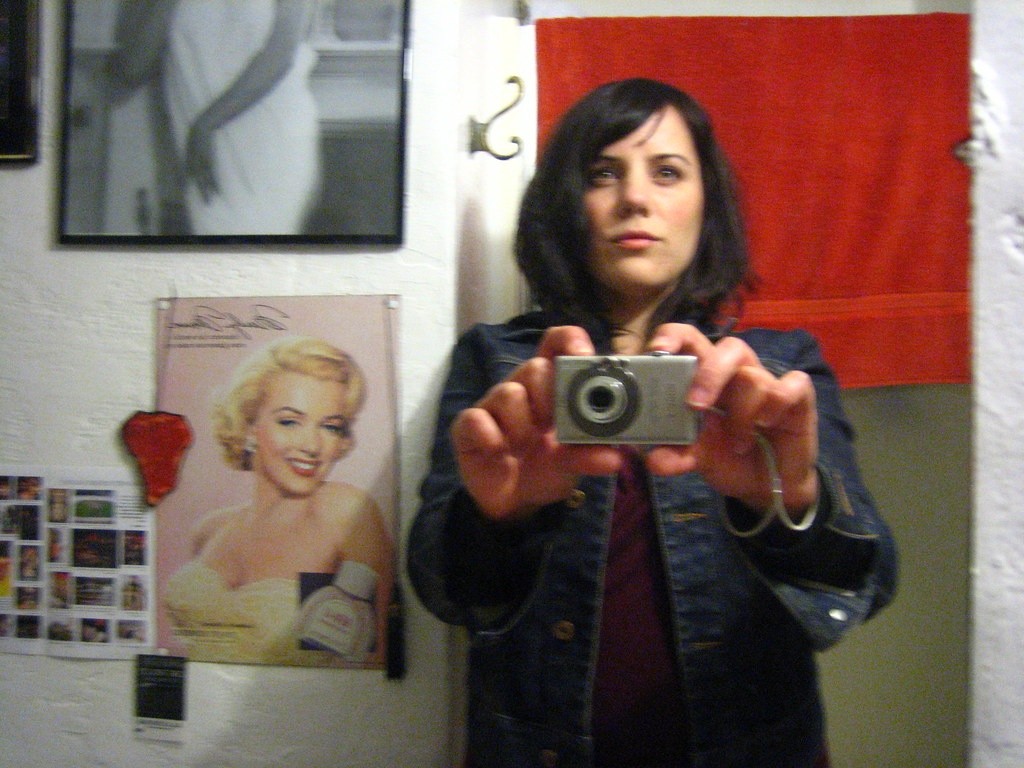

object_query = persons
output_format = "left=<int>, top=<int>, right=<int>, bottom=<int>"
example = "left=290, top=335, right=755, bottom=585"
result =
left=116, top=0, right=325, bottom=235
left=404, top=77, right=897, bottom=768
left=162, top=337, right=396, bottom=670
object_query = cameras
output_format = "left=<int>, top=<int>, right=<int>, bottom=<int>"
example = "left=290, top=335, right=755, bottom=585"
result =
left=553, top=350, right=699, bottom=445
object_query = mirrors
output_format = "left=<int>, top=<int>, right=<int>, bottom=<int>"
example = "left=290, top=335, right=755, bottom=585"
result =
left=0, top=2, right=975, bottom=767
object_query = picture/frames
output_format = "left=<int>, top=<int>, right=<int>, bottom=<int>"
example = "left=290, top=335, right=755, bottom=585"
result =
left=59, top=5, right=409, bottom=246
left=0, top=4, right=42, bottom=164
